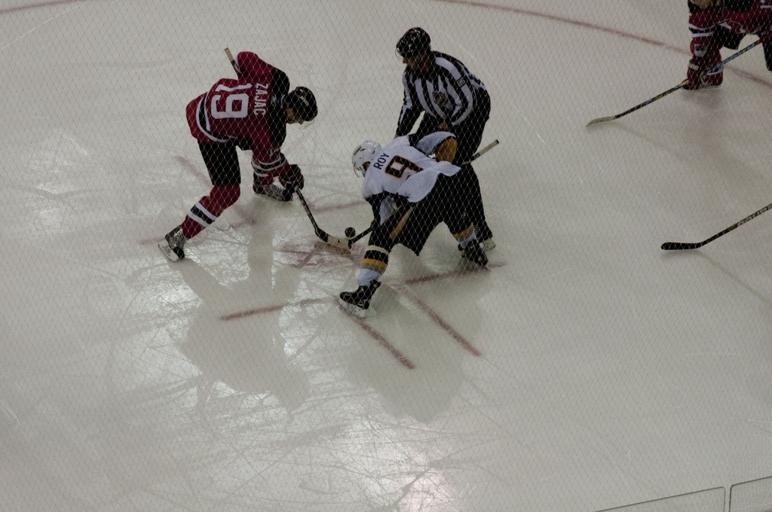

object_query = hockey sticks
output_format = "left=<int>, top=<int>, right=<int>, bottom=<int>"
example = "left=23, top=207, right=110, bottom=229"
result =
left=224, top=47, right=352, bottom=251
left=587, top=34, right=772, bottom=126
left=320, top=139, right=499, bottom=244
left=662, top=204, right=772, bottom=251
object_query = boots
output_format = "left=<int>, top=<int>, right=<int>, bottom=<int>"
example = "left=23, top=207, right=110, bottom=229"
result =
left=253, top=181, right=292, bottom=201
left=458, top=221, right=493, bottom=266
left=165, top=225, right=187, bottom=259
left=340, top=279, right=381, bottom=310
left=683, top=60, right=723, bottom=90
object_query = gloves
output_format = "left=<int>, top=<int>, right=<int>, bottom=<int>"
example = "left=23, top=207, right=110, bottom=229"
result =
left=687, top=58, right=713, bottom=81
left=279, top=164, right=304, bottom=193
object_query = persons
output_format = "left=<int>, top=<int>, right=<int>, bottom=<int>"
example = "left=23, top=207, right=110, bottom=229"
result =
left=163, top=51, right=318, bottom=259
left=392, top=26, right=493, bottom=252
left=683, top=1, right=772, bottom=89
left=339, top=130, right=488, bottom=310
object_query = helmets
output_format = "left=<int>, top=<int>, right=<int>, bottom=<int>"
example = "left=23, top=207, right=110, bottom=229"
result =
left=289, top=87, right=317, bottom=124
left=396, top=28, right=430, bottom=58
left=352, top=140, right=383, bottom=170
